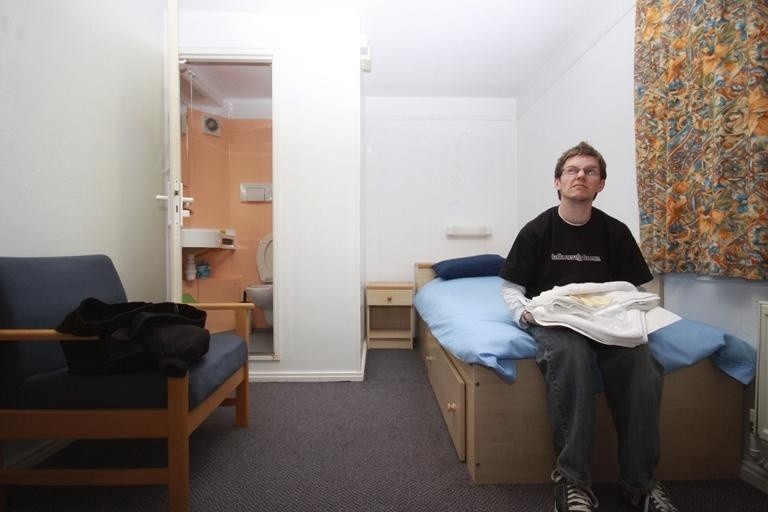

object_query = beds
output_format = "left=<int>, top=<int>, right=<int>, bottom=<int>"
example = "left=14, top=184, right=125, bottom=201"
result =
left=413, top=262, right=748, bottom=486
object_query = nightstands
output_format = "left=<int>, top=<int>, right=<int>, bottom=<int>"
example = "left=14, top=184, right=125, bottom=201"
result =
left=363, top=282, right=415, bottom=351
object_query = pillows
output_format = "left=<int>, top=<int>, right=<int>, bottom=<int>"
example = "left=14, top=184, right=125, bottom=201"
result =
left=431, top=253, right=508, bottom=280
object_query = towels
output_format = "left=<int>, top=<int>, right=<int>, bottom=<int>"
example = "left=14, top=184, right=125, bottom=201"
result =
left=521, top=276, right=680, bottom=352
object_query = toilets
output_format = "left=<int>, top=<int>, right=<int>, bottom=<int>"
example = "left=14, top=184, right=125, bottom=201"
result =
left=246, top=234, right=273, bottom=326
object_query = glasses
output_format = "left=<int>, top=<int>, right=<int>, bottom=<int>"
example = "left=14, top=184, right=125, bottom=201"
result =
left=560, top=165, right=599, bottom=176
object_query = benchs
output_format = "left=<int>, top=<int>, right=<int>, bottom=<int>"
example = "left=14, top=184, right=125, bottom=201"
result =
left=0, top=253, right=257, bottom=512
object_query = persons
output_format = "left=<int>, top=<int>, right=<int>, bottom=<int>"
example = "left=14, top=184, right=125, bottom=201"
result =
left=498, top=142, right=683, bottom=512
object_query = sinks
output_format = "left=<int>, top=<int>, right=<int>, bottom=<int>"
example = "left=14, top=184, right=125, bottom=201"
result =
left=182, top=229, right=238, bottom=249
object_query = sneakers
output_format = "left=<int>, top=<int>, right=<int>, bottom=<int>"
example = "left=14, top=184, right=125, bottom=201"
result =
left=625, top=481, right=679, bottom=511
left=552, top=467, right=599, bottom=512
